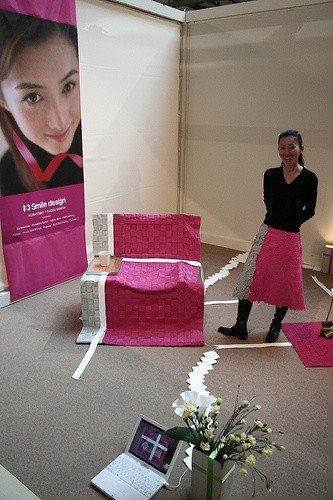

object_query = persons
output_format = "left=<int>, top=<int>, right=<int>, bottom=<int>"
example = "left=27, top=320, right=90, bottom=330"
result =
left=217, top=129, right=319, bottom=344
left=0, top=10, right=83, bottom=197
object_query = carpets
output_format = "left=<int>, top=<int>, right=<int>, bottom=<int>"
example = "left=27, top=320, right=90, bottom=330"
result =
left=281, top=321, right=333, bottom=368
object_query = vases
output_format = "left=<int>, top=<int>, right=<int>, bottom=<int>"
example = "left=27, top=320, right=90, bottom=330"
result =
left=191, top=446, right=235, bottom=500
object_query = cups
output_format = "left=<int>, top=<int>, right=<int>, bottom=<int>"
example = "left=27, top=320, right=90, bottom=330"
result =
left=98, top=251, right=111, bottom=266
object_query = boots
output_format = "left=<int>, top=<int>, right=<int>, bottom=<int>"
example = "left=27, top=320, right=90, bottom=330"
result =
left=265, top=305, right=288, bottom=343
left=217, top=299, right=253, bottom=340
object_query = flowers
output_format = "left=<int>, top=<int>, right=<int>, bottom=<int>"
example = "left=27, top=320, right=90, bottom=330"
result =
left=155, top=386, right=286, bottom=498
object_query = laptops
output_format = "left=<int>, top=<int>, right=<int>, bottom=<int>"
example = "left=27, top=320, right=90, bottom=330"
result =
left=90, top=414, right=184, bottom=500
